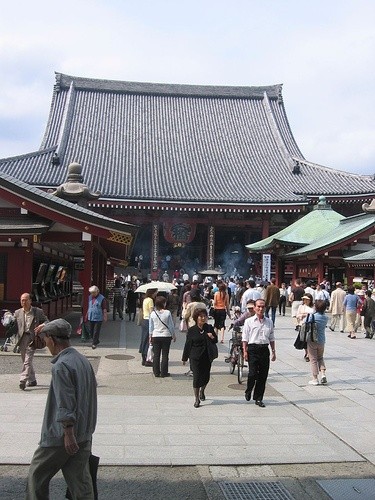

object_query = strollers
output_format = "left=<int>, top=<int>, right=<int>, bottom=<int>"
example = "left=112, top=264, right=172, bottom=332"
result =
left=0, top=309, right=20, bottom=353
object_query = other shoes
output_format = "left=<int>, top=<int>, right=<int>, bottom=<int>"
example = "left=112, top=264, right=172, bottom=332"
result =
left=348, top=335, right=356, bottom=339
left=194, top=401, right=200, bottom=408
left=304, top=353, right=310, bottom=362
left=92, top=345, right=96, bottom=349
left=142, top=361, right=153, bottom=367
left=18, top=380, right=37, bottom=390
left=200, top=390, right=205, bottom=401
left=365, top=333, right=374, bottom=339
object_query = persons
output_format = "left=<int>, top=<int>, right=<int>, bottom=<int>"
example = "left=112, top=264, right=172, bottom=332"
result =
left=139, top=288, right=158, bottom=366
left=27, top=318, right=98, bottom=500
left=182, top=308, right=218, bottom=408
left=294, top=293, right=315, bottom=361
left=301, top=300, right=329, bottom=385
left=11, top=292, right=49, bottom=390
left=241, top=299, right=276, bottom=408
left=110, top=252, right=334, bottom=334
left=182, top=288, right=209, bottom=376
left=210, top=284, right=233, bottom=343
left=84, top=285, right=107, bottom=349
left=353, top=283, right=375, bottom=339
left=343, top=286, right=363, bottom=339
left=148, top=296, right=176, bottom=377
left=329, top=282, right=346, bottom=333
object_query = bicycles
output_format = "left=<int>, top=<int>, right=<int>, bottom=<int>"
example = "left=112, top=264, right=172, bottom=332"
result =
left=225, top=323, right=248, bottom=384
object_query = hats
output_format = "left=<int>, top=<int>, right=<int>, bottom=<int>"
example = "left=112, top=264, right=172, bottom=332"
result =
left=302, top=293, right=313, bottom=301
left=40, top=318, right=71, bottom=337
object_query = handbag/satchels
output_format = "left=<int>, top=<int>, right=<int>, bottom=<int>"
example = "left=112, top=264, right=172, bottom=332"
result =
left=65, top=452, right=100, bottom=500
left=360, top=307, right=368, bottom=316
left=205, top=325, right=218, bottom=362
left=34, top=331, right=46, bottom=349
left=137, top=308, right=143, bottom=326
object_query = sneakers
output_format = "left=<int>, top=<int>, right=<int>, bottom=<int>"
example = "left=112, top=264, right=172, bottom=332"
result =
left=308, top=380, right=319, bottom=386
left=255, top=400, right=265, bottom=407
left=320, top=376, right=328, bottom=384
left=245, top=391, right=251, bottom=401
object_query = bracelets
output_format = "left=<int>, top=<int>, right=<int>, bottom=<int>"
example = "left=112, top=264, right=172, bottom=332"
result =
left=272, top=349, right=275, bottom=352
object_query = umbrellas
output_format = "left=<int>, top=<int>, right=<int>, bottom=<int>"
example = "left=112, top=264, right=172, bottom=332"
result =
left=134, top=281, right=178, bottom=293
